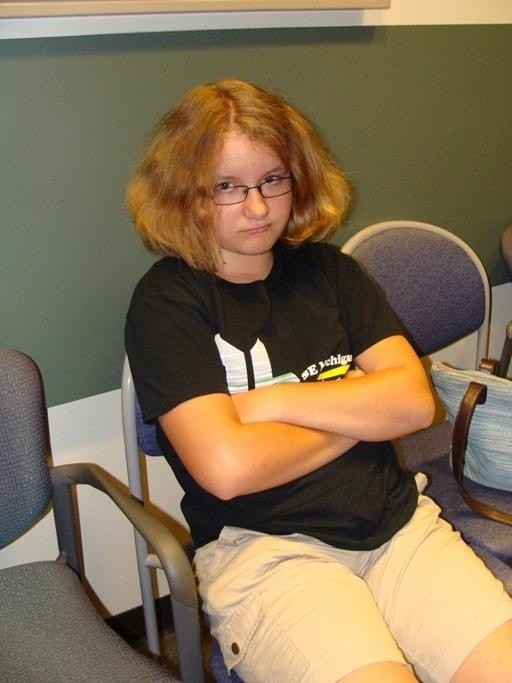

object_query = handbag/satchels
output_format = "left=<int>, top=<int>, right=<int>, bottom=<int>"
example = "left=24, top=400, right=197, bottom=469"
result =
left=430, top=362, right=512, bottom=528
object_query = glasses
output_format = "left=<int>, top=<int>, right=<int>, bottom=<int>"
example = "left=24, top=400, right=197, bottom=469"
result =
left=210, top=174, right=297, bottom=206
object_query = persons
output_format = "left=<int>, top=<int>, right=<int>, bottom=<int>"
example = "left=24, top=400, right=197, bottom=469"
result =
left=119, top=74, right=511, bottom=683
